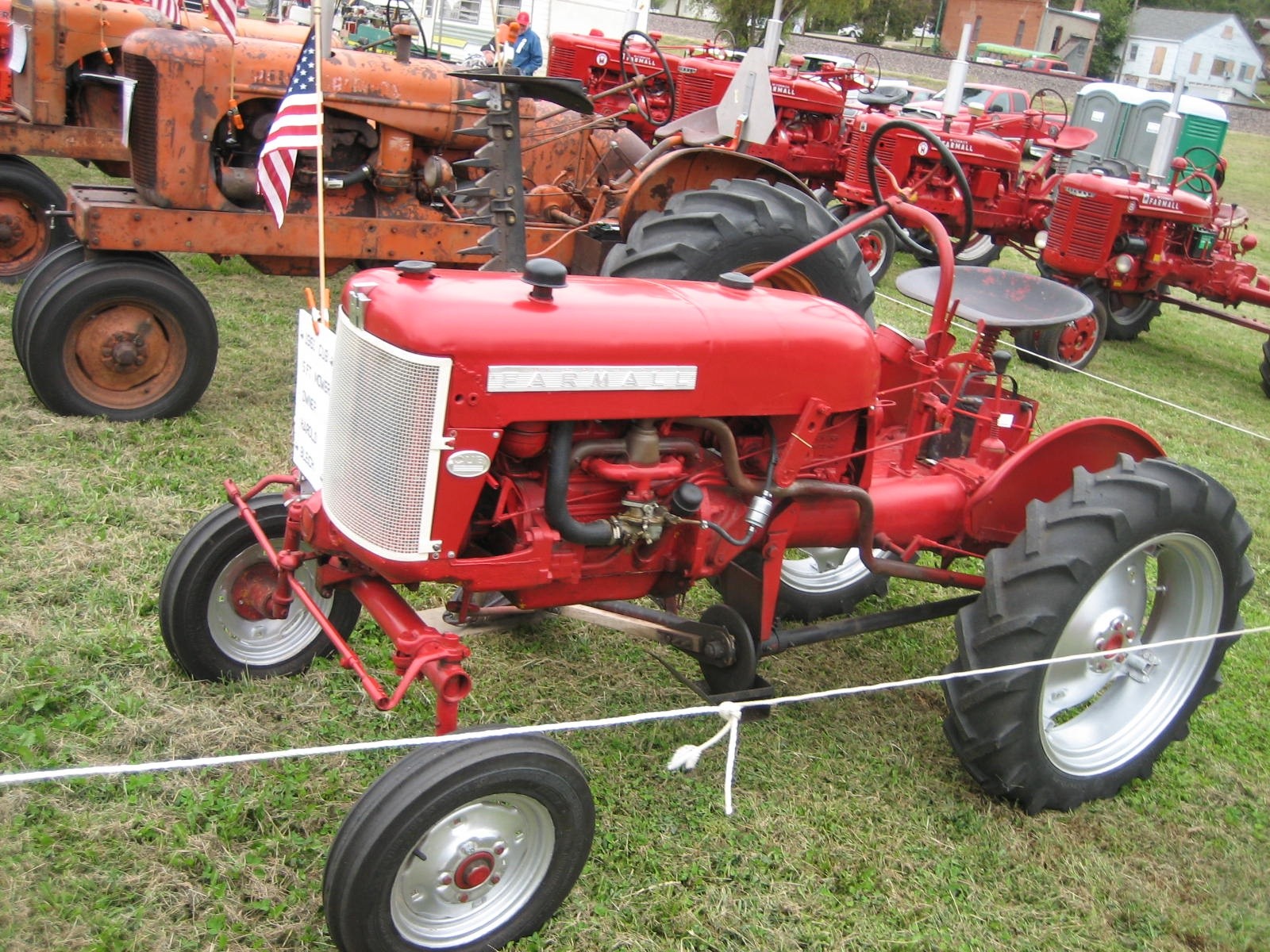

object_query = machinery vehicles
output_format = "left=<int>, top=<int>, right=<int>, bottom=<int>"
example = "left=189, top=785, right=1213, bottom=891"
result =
left=154, top=72, right=1249, bottom=950
left=0, top=0, right=1270, bottom=427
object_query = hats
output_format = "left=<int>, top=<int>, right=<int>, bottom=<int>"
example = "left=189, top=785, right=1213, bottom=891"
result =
left=517, top=11, right=530, bottom=26
left=507, top=22, right=520, bottom=44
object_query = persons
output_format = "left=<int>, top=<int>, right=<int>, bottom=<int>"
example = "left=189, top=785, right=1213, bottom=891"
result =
left=482, top=12, right=542, bottom=76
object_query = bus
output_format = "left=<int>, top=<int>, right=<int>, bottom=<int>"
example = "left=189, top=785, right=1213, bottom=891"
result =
left=971, top=42, right=1063, bottom=68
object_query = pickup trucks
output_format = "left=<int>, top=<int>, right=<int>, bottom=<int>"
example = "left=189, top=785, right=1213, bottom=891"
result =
left=1004, top=56, right=1104, bottom=83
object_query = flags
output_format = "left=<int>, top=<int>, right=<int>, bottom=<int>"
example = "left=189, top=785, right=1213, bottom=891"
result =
left=150, top=0, right=181, bottom=24
left=209, top=0, right=236, bottom=42
left=256, top=27, right=324, bottom=228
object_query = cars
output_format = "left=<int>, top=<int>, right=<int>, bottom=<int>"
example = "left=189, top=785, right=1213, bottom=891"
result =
left=837, top=25, right=863, bottom=39
